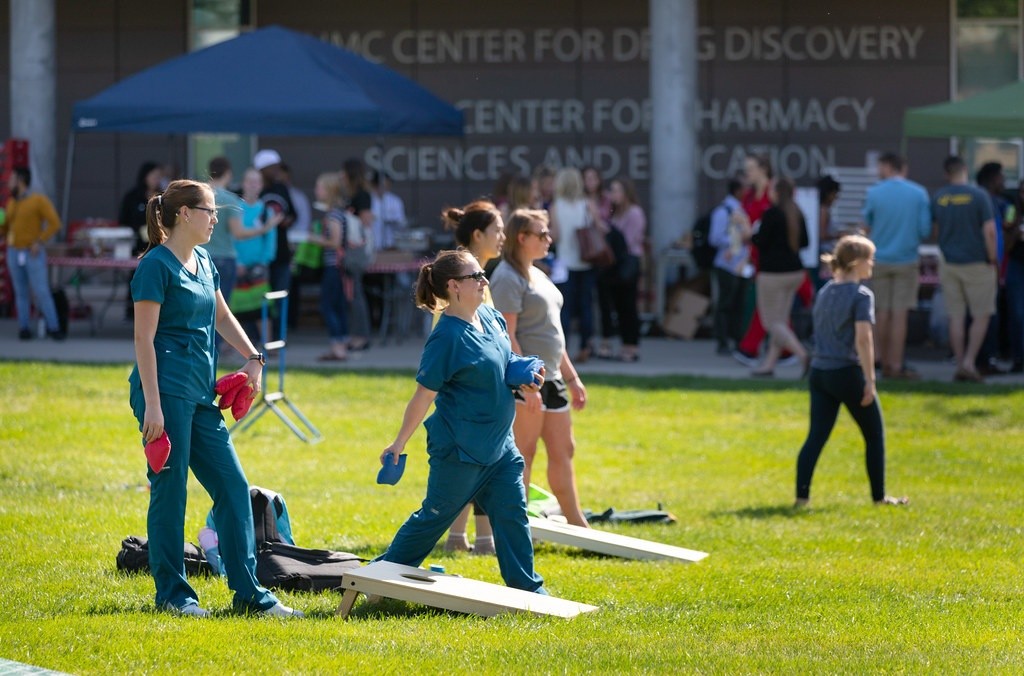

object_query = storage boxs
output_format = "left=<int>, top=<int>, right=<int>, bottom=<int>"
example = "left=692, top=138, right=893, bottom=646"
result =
left=661, top=289, right=710, bottom=339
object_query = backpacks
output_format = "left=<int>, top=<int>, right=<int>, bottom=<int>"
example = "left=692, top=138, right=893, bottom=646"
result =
left=692, top=203, right=732, bottom=268
left=256, top=539, right=370, bottom=595
left=328, top=208, right=367, bottom=277
left=116, top=536, right=214, bottom=577
left=199, top=485, right=296, bottom=579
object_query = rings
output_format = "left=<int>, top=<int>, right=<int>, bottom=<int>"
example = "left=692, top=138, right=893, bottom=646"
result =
left=148, top=429, right=153, bottom=432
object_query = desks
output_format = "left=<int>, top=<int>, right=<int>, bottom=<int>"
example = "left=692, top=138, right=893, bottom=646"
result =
left=354, top=251, right=434, bottom=346
left=46, top=256, right=148, bottom=337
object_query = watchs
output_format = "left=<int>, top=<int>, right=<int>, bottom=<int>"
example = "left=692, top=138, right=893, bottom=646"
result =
left=248, top=353, right=266, bottom=366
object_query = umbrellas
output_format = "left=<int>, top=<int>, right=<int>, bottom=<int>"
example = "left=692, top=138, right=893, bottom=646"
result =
left=67, top=29, right=465, bottom=173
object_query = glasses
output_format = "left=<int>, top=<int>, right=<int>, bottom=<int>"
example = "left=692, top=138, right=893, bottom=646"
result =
left=191, top=206, right=218, bottom=217
left=528, top=231, right=550, bottom=241
left=449, top=270, right=486, bottom=280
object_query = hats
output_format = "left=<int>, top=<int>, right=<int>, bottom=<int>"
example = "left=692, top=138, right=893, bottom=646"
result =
left=939, top=154, right=966, bottom=180
left=252, top=149, right=281, bottom=171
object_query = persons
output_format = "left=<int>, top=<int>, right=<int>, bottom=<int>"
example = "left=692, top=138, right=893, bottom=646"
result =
left=492, top=165, right=646, bottom=364
left=793, top=235, right=910, bottom=510
left=129, top=180, right=309, bottom=620
left=361, top=202, right=589, bottom=603
left=122, top=151, right=408, bottom=364
left=710, top=152, right=1024, bottom=384
left=4, top=165, right=63, bottom=341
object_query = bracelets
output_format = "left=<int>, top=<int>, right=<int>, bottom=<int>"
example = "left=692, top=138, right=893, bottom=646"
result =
left=989, top=258, right=997, bottom=264
left=567, top=375, right=579, bottom=384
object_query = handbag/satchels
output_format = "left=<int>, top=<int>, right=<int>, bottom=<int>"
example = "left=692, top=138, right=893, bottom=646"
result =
left=293, top=220, right=322, bottom=277
left=576, top=228, right=614, bottom=265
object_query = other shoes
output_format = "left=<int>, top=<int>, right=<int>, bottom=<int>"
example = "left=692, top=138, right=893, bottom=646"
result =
left=316, top=350, right=347, bottom=363
left=800, top=354, right=811, bottom=379
left=732, top=349, right=761, bottom=368
left=536, top=587, right=549, bottom=596
left=343, top=339, right=371, bottom=353
left=614, top=353, right=640, bottom=363
left=596, top=347, right=614, bottom=359
left=874, top=344, right=1017, bottom=386
left=263, top=601, right=306, bottom=619
left=748, top=370, right=774, bottom=379
left=573, top=347, right=592, bottom=363
left=875, top=495, right=911, bottom=508
left=776, top=352, right=797, bottom=368
left=177, top=603, right=210, bottom=617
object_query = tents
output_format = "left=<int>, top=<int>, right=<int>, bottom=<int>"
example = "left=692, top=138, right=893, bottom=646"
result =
left=897, top=82, right=1024, bottom=183
